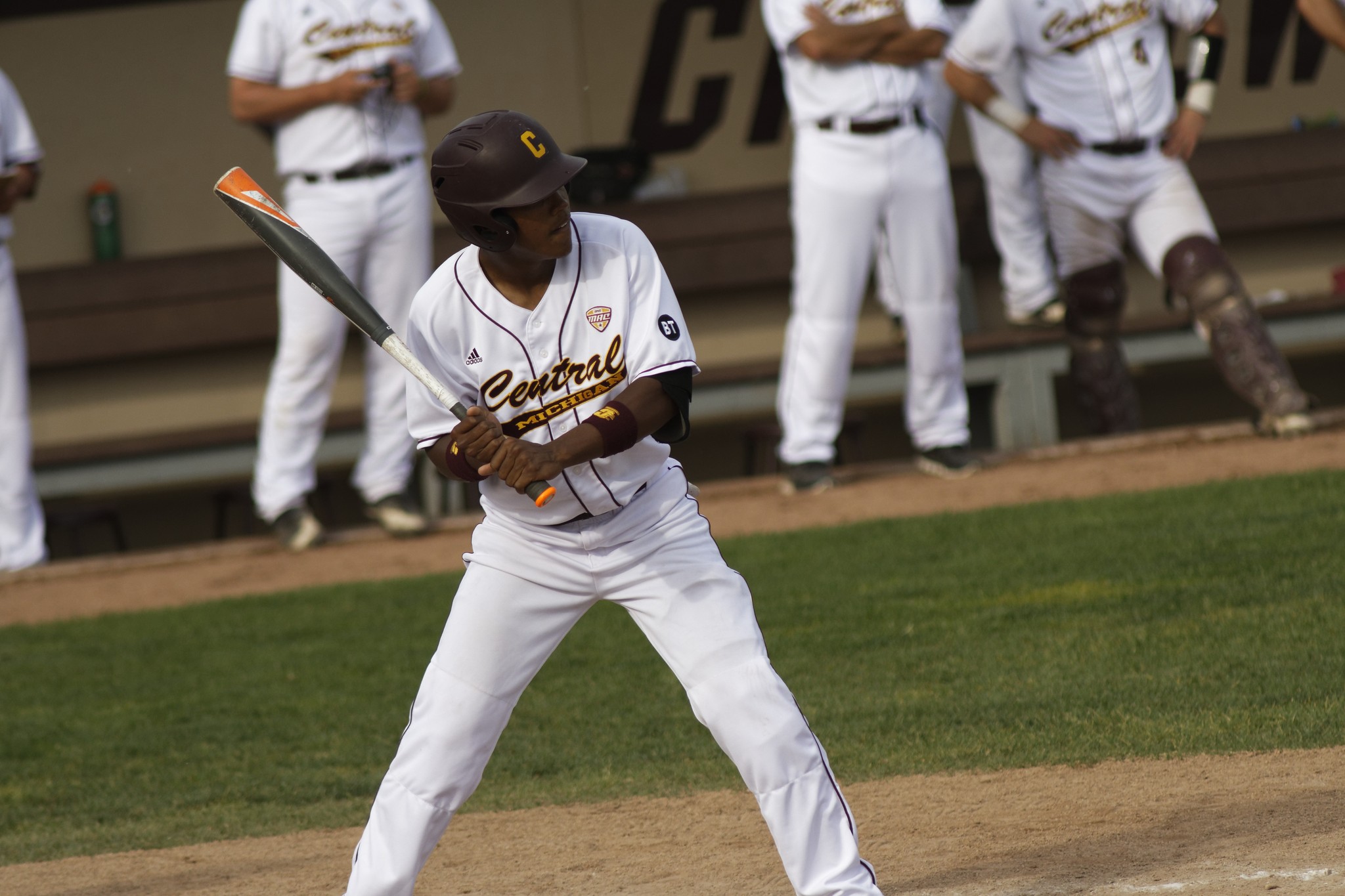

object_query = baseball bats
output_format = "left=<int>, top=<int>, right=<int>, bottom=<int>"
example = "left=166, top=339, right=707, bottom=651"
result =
left=210, top=166, right=557, bottom=509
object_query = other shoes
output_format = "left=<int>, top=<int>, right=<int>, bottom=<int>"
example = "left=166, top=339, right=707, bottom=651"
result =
left=369, top=488, right=430, bottom=536
left=781, top=462, right=829, bottom=500
left=1007, top=292, right=1069, bottom=329
left=913, top=440, right=972, bottom=480
left=274, top=509, right=323, bottom=548
left=1257, top=388, right=1311, bottom=435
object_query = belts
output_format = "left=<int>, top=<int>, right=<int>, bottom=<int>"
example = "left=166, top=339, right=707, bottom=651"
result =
left=820, top=108, right=925, bottom=134
left=1098, top=139, right=1148, bottom=156
left=304, top=154, right=421, bottom=183
left=550, top=480, right=648, bottom=526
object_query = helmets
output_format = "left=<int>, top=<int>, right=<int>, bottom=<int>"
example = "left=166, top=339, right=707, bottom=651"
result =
left=429, top=108, right=592, bottom=253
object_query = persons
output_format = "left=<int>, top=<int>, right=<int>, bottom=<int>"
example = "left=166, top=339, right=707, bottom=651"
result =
left=344, top=109, right=882, bottom=896
left=0, top=67, right=47, bottom=576
left=224, top=0, right=465, bottom=551
left=1297, top=0, right=1345, bottom=49
left=874, top=0, right=1318, bottom=439
left=761, top=0, right=981, bottom=498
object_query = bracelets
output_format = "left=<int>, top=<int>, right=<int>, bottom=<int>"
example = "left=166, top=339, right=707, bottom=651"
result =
left=445, top=440, right=490, bottom=482
left=581, top=400, right=638, bottom=458
